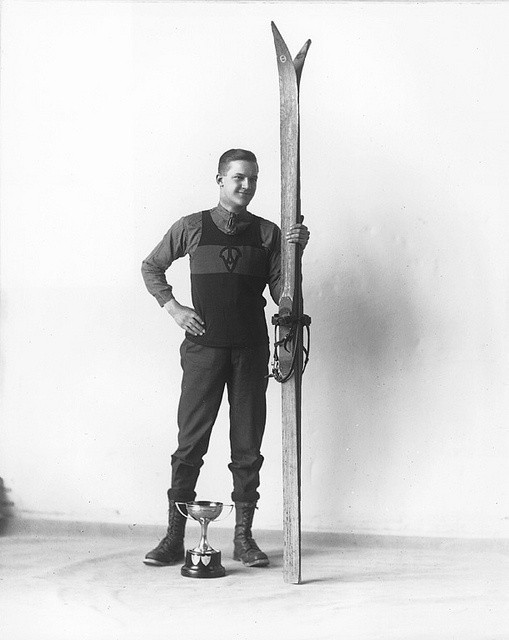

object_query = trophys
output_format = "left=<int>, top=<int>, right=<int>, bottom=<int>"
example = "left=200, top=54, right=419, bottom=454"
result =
left=175, top=501, right=234, bottom=578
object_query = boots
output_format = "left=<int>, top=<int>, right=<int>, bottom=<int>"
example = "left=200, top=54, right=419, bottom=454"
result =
left=143, top=488, right=196, bottom=566
left=231, top=492, right=269, bottom=567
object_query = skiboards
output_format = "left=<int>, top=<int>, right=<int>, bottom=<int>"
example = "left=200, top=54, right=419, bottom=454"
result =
left=271, top=20, right=311, bottom=584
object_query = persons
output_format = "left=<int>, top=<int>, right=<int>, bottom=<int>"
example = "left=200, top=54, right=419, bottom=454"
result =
left=142, top=149, right=310, bottom=567
